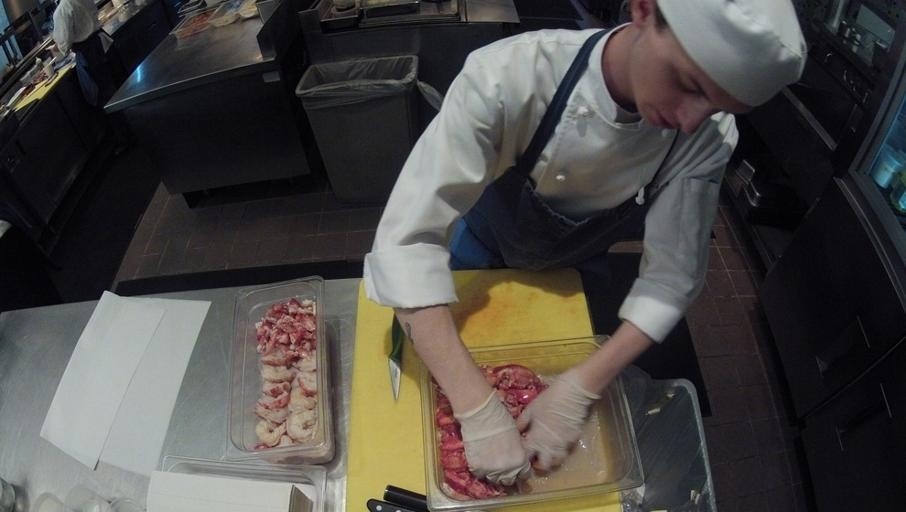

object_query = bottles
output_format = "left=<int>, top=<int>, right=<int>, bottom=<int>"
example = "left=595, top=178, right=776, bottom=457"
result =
left=35, top=56, right=44, bottom=70
left=93, top=2, right=119, bottom=22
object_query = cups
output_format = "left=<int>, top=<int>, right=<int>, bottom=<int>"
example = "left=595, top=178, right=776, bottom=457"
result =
left=41, top=64, right=55, bottom=78
left=0, top=479, right=144, bottom=511
left=838, top=20, right=864, bottom=53
left=871, top=143, right=906, bottom=216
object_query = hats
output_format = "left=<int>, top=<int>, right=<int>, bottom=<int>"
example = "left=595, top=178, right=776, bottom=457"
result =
left=653, top=1, right=809, bottom=110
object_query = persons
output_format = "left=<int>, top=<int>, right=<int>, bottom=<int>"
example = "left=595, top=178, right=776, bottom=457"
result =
left=356, top=1, right=807, bottom=488
left=49, top=0, right=133, bottom=146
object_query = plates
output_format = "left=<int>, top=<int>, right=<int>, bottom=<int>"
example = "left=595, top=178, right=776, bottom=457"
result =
left=4, top=86, right=28, bottom=108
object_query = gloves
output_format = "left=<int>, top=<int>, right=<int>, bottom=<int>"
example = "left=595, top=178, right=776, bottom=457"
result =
left=451, top=386, right=534, bottom=487
left=515, top=367, right=604, bottom=474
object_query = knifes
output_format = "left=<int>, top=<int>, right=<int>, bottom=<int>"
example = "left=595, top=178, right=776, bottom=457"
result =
left=386, top=317, right=405, bottom=400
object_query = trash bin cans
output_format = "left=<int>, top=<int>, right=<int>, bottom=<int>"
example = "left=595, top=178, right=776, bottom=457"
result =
left=602, top=379, right=718, bottom=512
left=295, top=54, right=422, bottom=204
